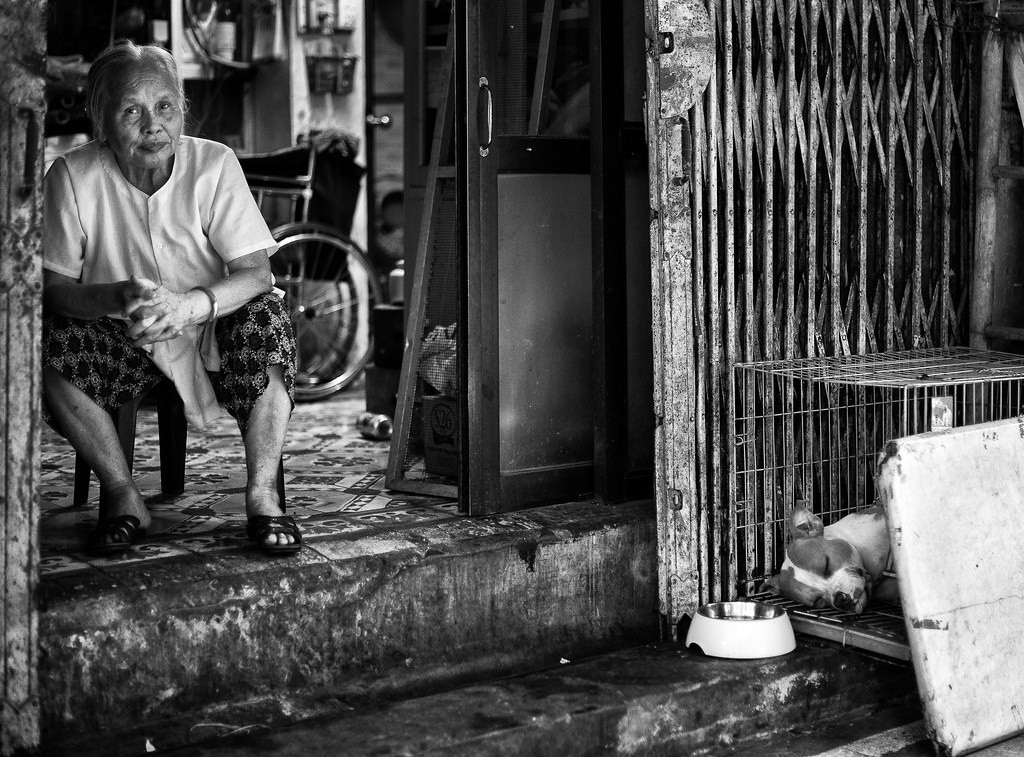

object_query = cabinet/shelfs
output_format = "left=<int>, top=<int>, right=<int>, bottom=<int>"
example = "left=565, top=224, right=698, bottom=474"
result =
left=108, top=0, right=256, bottom=157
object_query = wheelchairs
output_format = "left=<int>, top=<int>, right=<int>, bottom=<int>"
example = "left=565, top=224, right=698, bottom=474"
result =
left=233, top=125, right=384, bottom=407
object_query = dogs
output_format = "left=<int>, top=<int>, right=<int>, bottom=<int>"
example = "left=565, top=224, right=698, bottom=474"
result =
left=763, top=495, right=894, bottom=621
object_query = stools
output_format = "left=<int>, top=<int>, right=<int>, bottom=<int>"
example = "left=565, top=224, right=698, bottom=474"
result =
left=74, top=390, right=288, bottom=513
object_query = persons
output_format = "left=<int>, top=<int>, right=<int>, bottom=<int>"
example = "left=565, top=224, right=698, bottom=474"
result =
left=42, top=38, right=305, bottom=555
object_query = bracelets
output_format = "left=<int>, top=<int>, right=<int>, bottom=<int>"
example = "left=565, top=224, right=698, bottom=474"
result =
left=190, top=286, right=218, bottom=327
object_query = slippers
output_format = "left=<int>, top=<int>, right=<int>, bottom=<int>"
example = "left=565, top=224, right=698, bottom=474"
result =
left=246, top=513, right=304, bottom=553
left=89, top=514, right=148, bottom=555
